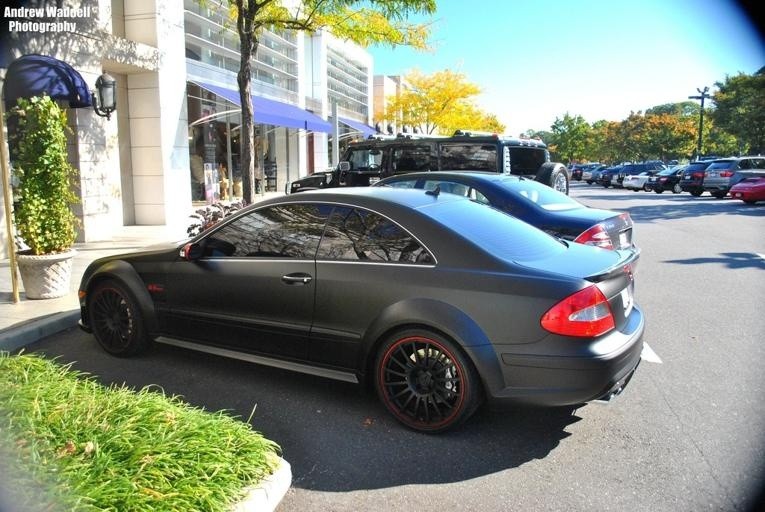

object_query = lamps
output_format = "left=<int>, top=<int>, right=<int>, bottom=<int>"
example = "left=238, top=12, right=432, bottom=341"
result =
left=87, top=68, right=117, bottom=121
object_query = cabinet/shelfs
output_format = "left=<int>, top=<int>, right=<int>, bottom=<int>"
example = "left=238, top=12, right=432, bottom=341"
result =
left=256, top=157, right=278, bottom=192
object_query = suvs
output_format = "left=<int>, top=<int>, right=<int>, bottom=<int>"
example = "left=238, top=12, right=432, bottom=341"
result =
left=284, top=130, right=569, bottom=198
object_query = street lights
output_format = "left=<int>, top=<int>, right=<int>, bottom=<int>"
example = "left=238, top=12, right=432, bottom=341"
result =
left=688, top=86, right=714, bottom=157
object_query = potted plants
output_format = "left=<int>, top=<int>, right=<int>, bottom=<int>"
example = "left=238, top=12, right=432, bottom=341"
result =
left=3, top=92, right=80, bottom=300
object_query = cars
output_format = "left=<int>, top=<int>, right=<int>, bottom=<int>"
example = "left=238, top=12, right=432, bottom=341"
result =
left=75, top=185, right=645, bottom=434
left=369, top=169, right=637, bottom=253
left=571, top=155, right=765, bottom=206
